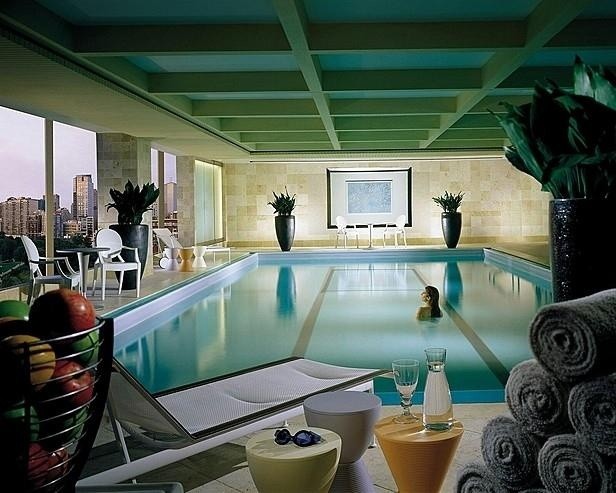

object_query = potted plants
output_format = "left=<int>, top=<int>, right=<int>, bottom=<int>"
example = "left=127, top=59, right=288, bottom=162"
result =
left=105, top=177, right=160, bottom=290
left=432, top=191, right=467, bottom=248
left=485, top=53, right=616, bottom=302
left=267, top=184, right=298, bottom=250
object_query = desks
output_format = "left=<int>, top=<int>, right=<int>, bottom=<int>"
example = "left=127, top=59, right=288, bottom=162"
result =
left=358, top=223, right=383, bottom=249
left=56, top=247, right=109, bottom=311
left=206, top=247, right=232, bottom=265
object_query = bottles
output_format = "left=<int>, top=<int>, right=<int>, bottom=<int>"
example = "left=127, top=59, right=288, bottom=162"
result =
left=423, top=348, right=454, bottom=431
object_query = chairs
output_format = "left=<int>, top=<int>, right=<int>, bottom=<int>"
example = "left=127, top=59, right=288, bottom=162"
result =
left=92, top=228, right=141, bottom=300
left=384, top=215, right=408, bottom=247
left=21, top=234, right=83, bottom=306
left=153, top=228, right=194, bottom=266
left=76, top=356, right=395, bottom=493
left=335, top=216, right=358, bottom=248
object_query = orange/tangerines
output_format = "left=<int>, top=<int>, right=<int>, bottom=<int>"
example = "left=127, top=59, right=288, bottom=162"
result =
left=0, top=398, right=40, bottom=444
left=67, top=330, right=100, bottom=372
left=41, top=405, right=88, bottom=451
left=0, top=300, right=29, bottom=321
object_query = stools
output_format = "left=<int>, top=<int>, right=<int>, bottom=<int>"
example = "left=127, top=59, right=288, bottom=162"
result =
left=162, top=246, right=207, bottom=271
left=245, top=428, right=343, bottom=493
left=304, top=390, right=382, bottom=493
left=372, top=413, right=464, bottom=493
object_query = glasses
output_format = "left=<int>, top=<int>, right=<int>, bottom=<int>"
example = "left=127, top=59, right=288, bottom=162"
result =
left=274, top=428, right=321, bottom=447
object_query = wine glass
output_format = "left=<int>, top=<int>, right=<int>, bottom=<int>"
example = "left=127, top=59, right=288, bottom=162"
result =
left=391, top=360, right=421, bottom=426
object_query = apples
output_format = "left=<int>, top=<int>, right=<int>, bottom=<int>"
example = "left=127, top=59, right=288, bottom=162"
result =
left=29, top=289, right=96, bottom=346
left=16, top=442, right=49, bottom=493
left=33, top=360, right=93, bottom=412
left=48, top=445, right=69, bottom=482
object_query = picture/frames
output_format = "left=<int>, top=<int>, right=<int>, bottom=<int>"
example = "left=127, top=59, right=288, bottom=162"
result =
left=326, top=167, right=413, bottom=228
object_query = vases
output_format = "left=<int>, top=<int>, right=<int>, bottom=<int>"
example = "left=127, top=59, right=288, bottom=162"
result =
left=273, top=265, right=298, bottom=323
left=442, top=260, right=466, bottom=312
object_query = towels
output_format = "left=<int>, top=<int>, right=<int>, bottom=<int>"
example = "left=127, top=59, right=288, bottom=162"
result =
left=445, top=287, right=615, bottom=492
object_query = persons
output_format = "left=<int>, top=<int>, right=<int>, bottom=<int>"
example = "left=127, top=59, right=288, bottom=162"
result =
left=415, top=285, right=444, bottom=319
